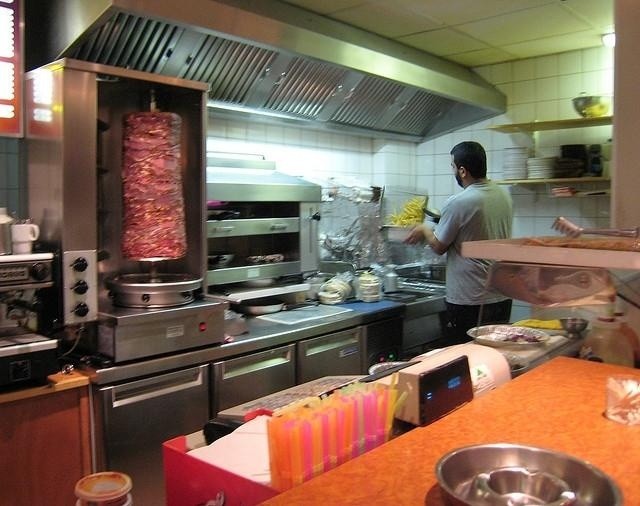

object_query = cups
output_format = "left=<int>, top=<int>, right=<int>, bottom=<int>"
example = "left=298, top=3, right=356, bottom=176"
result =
left=74, top=472, right=135, bottom=506
left=10, top=222, right=40, bottom=255
left=605, top=374, right=640, bottom=425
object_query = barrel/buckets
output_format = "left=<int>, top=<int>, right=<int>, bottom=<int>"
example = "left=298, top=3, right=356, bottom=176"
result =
left=580, top=316, right=634, bottom=368
left=611, top=311, right=640, bottom=361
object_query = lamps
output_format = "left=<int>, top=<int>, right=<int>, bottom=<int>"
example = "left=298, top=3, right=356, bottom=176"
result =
left=601, top=24, right=616, bottom=49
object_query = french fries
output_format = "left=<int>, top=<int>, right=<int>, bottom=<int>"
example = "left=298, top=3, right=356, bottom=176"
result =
left=389, top=197, right=424, bottom=226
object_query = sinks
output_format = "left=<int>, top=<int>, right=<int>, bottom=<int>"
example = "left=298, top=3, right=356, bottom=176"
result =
left=394, top=265, right=447, bottom=285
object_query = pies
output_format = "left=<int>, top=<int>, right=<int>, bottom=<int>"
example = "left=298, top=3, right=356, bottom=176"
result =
left=318, top=272, right=379, bottom=304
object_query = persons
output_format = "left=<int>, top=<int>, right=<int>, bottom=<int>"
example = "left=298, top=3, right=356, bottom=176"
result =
left=402, top=141, right=514, bottom=342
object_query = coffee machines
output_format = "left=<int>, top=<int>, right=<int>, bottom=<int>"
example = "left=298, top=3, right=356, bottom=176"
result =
left=0, top=248, right=59, bottom=392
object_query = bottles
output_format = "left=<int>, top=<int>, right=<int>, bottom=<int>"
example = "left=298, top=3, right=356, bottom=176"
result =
left=1, top=206, right=14, bottom=256
left=383, top=264, right=399, bottom=294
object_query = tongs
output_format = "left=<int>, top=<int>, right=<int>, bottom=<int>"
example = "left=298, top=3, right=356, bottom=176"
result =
left=550, top=214, right=638, bottom=239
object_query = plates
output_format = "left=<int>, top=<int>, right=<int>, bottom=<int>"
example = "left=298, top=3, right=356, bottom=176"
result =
left=466, top=322, right=551, bottom=349
left=239, top=300, right=284, bottom=316
left=368, top=361, right=409, bottom=376
left=502, top=141, right=588, bottom=181
left=243, top=279, right=274, bottom=288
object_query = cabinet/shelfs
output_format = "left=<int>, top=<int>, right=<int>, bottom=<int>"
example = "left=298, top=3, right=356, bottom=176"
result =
left=489, top=115, right=613, bottom=191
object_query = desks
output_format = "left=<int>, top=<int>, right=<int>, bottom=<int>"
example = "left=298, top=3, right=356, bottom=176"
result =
left=256, top=357, right=640, bottom=505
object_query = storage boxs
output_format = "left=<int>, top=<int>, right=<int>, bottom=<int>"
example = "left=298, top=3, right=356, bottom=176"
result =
left=162, top=430, right=279, bottom=505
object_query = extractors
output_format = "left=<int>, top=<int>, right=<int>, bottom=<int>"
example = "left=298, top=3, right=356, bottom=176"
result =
left=28, top=0, right=509, bottom=144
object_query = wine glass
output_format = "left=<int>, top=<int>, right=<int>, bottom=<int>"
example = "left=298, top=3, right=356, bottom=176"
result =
left=560, top=317, right=590, bottom=339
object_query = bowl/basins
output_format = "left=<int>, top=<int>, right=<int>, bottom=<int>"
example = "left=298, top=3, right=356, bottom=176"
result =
left=432, top=439, right=624, bottom=505
left=571, top=95, right=611, bottom=117
left=207, top=252, right=236, bottom=266
